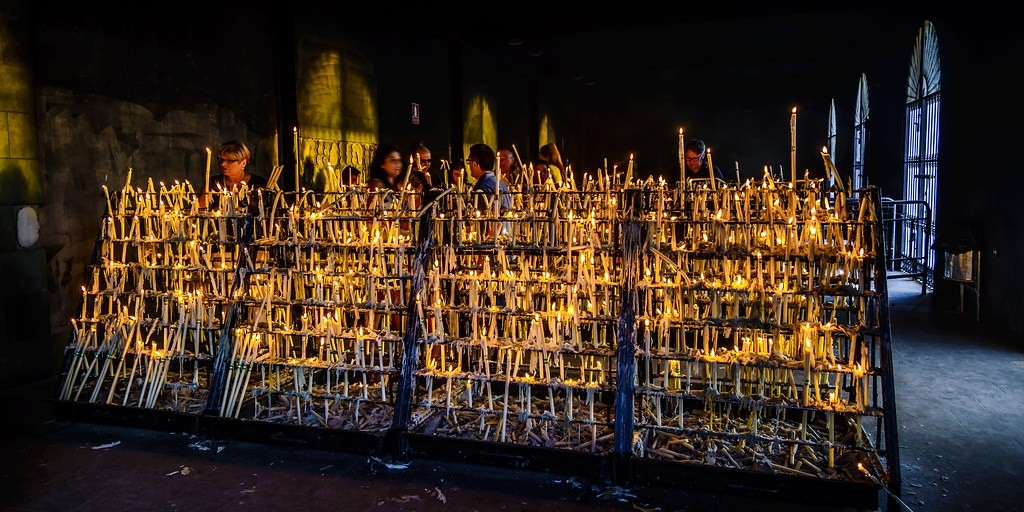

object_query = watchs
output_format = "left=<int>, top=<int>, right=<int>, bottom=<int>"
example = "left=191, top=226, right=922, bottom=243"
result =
left=477, top=254, right=485, bottom=260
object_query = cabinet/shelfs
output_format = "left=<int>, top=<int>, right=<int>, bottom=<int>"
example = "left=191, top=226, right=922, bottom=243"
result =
left=53, top=192, right=902, bottom=512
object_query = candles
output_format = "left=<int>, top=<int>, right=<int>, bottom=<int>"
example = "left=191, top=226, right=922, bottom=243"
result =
left=60, top=106, right=874, bottom=475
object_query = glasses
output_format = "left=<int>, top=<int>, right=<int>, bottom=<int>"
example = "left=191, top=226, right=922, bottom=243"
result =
left=685, top=154, right=702, bottom=162
left=216, top=157, right=238, bottom=164
left=466, top=159, right=479, bottom=165
left=421, top=159, right=431, bottom=163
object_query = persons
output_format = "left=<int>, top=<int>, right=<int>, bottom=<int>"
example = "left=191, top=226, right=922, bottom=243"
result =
left=540, top=141, right=571, bottom=190
left=198, top=139, right=271, bottom=208
left=684, top=136, right=723, bottom=179
left=491, top=147, right=519, bottom=185
left=530, top=158, right=549, bottom=182
left=332, top=142, right=470, bottom=370
left=466, top=142, right=515, bottom=366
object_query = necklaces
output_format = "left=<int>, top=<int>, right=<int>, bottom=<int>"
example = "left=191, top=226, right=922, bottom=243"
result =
left=225, top=172, right=247, bottom=188
